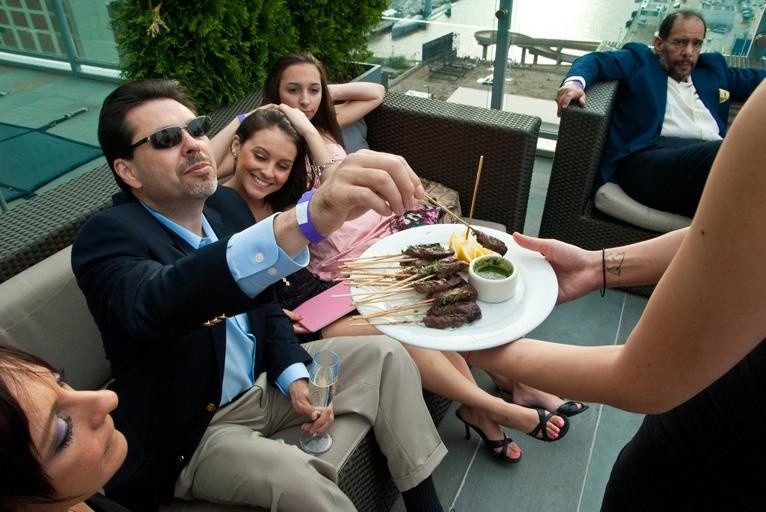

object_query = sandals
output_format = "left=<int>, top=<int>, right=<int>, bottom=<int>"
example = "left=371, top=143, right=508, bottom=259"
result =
left=555, top=401, right=590, bottom=417
left=525, top=407, right=571, bottom=443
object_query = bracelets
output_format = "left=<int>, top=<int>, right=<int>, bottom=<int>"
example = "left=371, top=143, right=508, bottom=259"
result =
left=307, top=155, right=344, bottom=177
left=564, top=81, right=584, bottom=90
left=295, top=190, right=326, bottom=247
left=601, top=246, right=608, bottom=297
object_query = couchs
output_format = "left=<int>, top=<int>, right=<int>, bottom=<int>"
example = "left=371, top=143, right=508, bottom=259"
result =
left=534, top=48, right=765, bottom=302
left=1, top=64, right=545, bottom=510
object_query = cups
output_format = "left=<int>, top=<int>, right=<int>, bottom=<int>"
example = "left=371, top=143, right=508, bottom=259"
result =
left=468, top=251, right=518, bottom=305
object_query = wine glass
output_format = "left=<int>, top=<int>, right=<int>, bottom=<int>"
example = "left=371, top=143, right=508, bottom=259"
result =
left=299, top=349, right=340, bottom=455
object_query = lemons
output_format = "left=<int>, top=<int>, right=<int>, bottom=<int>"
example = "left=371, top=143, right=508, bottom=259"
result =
left=448, top=233, right=490, bottom=263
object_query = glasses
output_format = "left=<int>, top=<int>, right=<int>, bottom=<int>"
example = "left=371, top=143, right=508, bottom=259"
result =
left=130, top=115, right=213, bottom=151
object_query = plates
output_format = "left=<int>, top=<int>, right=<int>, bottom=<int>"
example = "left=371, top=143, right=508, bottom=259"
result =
left=350, top=225, right=559, bottom=354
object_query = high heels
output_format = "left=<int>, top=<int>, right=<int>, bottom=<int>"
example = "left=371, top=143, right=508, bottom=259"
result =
left=455, top=409, right=522, bottom=463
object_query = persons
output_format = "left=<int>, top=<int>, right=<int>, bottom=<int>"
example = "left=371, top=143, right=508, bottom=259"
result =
left=205, top=49, right=592, bottom=418
left=65, top=71, right=451, bottom=511
left=447, top=68, right=765, bottom=511
left=227, top=103, right=572, bottom=466
left=550, top=7, right=766, bottom=220
left=1, top=342, right=131, bottom=510
